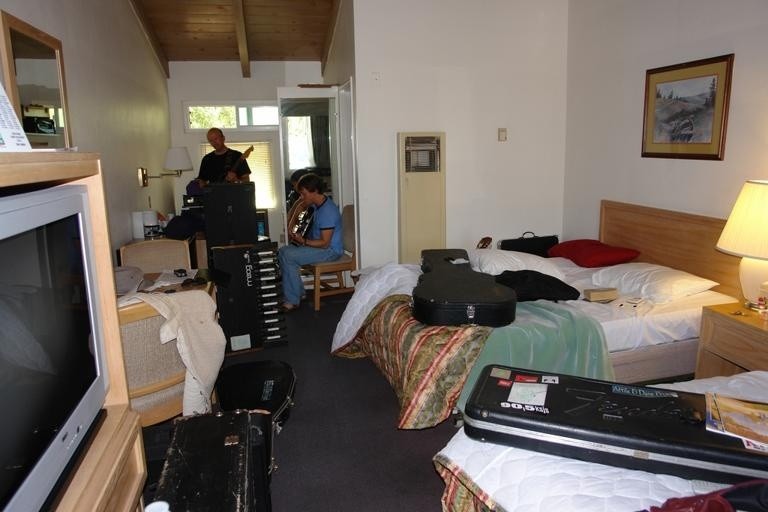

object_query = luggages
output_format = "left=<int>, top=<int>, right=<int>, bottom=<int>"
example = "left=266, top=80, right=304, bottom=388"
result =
left=152, top=408, right=275, bottom=511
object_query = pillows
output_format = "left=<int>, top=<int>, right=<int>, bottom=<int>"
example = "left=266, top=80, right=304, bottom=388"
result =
left=546, top=239, right=641, bottom=267
left=593, top=261, right=721, bottom=305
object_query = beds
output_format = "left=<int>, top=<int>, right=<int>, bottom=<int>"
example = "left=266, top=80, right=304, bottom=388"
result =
left=435, top=368, right=768, bottom=512
left=331, top=200, right=750, bottom=431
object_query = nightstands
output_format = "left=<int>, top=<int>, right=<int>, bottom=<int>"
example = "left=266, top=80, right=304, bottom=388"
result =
left=694, top=306, right=768, bottom=386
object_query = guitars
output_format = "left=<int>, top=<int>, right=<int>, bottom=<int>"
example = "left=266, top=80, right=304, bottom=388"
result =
left=291, top=207, right=315, bottom=246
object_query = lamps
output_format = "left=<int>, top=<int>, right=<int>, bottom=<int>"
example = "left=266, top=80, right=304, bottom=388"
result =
left=715, top=180, right=768, bottom=315
left=138, top=145, right=194, bottom=187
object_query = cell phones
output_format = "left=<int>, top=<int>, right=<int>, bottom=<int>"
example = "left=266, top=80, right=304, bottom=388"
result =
left=174, top=269, right=187, bottom=276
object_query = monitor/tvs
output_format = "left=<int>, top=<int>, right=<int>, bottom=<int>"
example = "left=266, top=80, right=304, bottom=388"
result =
left=0, top=185, right=111, bottom=512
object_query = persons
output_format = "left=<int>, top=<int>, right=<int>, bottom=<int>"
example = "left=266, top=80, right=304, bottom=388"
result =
left=278, top=169, right=344, bottom=313
left=199, top=128, right=251, bottom=187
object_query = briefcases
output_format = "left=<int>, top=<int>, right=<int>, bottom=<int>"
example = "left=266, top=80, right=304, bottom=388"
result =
left=496, top=230, right=560, bottom=257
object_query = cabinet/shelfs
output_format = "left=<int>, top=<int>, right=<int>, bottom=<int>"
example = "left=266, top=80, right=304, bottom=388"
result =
left=0, top=154, right=150, bottom=511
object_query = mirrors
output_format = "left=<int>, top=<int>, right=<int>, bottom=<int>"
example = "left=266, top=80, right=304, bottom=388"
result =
left=278, top=96, right=342, bottom=246
left=0, top=11, right=72, bottom=151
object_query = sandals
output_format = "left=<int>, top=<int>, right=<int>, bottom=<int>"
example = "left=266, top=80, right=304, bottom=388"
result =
left=277, top=295, right=306, bottom=313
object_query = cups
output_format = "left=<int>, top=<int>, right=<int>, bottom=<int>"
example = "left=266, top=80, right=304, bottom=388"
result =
left=130, top=209, right=160, bottom=240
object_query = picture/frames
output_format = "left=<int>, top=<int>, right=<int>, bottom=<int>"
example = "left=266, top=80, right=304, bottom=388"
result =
left=641, top=53, right=735, bottom=161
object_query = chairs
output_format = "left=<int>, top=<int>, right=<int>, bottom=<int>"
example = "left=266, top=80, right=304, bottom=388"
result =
left=117, top=299, right=224, bottom=428
left=298, top=204, right=359, bottom=311
left=120, top=240, right=192, bottom=277
